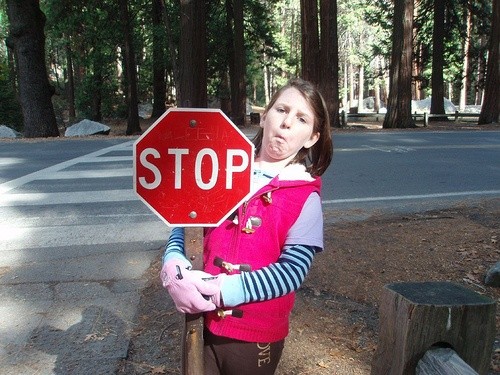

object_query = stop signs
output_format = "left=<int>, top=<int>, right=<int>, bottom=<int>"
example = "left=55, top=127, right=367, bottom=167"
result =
left=134, top=108, right=256, bottom=227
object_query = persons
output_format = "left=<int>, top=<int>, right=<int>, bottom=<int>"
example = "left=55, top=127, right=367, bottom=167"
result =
left=160, top=81, right=334, bottom=372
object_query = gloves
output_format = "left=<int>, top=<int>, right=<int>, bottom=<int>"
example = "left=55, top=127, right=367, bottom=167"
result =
left=188, top=268, right=226, bottom=309
left=160, top=257, right=216, bottom=314
